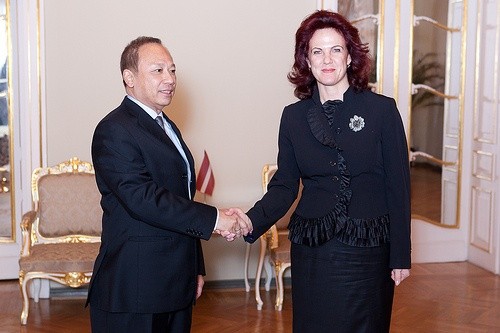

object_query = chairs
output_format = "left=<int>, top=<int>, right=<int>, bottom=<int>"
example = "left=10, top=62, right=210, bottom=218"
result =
left=262, top=164, right=304, bottom=311
left=18, top=157, right=104, bottom=326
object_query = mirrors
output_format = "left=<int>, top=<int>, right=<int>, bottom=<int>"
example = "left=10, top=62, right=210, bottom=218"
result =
left=0, top=0, right=17, bottom=243
left=336, top=0, right=381, bottom=94
left=408, top=0, right=468, bottom=228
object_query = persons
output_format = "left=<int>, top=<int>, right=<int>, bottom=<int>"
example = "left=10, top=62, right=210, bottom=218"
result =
left=212, top=10, right=411, bottom=333
left=84, top=36, right=249, bottom=332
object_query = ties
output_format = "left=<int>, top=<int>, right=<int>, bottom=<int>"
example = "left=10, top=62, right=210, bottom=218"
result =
left=155, top=116, right=165, bottom=132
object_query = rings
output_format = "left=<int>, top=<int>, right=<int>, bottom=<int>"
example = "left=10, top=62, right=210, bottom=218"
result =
left=234, top=228, right=241, bottom=234
